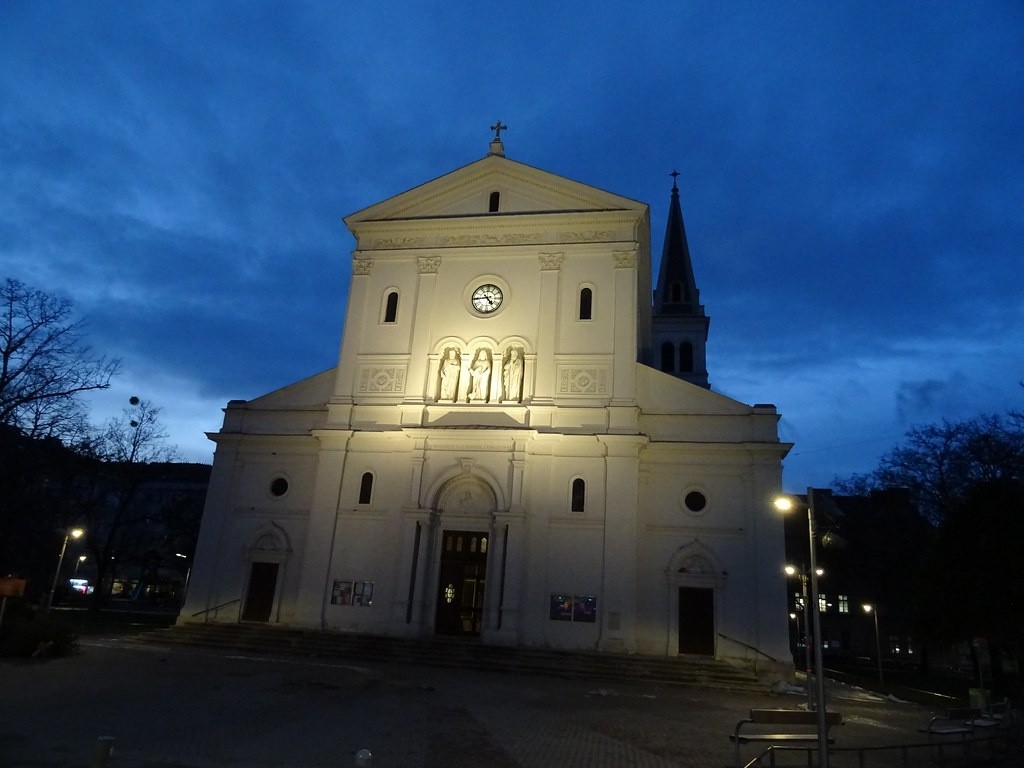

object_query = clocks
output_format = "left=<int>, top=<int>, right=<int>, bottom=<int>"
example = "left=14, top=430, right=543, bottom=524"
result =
left=472, top=284, right=503, bottom=313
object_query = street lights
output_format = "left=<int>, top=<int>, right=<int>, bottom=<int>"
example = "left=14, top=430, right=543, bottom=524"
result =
left=863, top=603, right=883, bottom=685
left=767, top=487, right=828, bottom=768
left=782, top=564, right=813, bottom=710
left=47, top=527, right=84, bottom=616
left=789, top=613, right=801, bottom=670
left=74, top=555, right=87, bottom=579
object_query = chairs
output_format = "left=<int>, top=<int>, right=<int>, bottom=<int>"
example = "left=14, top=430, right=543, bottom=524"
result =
left=729, top=708, right=845, bottom=768
left=917, top=697, right=1008, bottom=767
